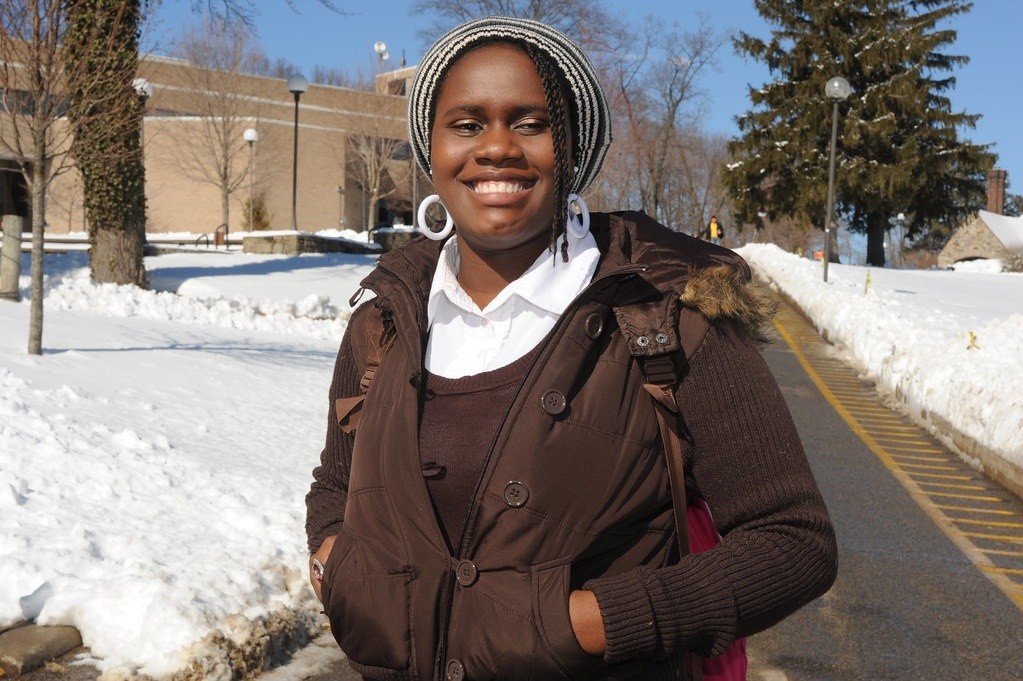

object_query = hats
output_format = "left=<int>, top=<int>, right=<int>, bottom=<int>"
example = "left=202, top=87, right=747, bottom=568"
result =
left=406, top=15, right=613, bottom=208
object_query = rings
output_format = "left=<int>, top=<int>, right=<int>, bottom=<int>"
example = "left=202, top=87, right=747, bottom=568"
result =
left=311, top=559, right=325, bottom=580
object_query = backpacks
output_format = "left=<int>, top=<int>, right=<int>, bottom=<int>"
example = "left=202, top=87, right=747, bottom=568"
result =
left=335, top=286, right=749, bottom=681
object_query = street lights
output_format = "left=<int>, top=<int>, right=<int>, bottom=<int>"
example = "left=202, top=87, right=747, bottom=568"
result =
left=243, top=127, right=259, bottom=234
left=287, top=73, right=308, bottom=229
left=824, top=74, right=851, bottom=281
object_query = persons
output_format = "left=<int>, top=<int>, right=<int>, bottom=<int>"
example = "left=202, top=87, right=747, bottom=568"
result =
left=705, top=216, right=723, bottom=246
left=304, top=15, right=839, bottom=681
